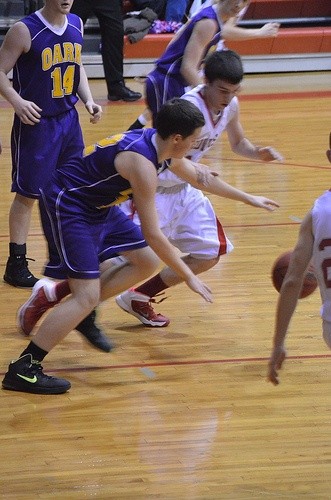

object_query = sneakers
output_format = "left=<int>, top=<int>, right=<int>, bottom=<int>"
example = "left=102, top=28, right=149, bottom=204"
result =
left=18, top=278, right=60, bottom=337
left=3, top=354, right=71, bottom=393
left=75, top=310, right=111, bottom=351
left=3, top=257, right=39, bottom=286
left=116, top=288, right=170, bottom=327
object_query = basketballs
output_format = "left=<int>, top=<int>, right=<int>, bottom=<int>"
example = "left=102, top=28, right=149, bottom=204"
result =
left=272, top=251, right=322, bottom=300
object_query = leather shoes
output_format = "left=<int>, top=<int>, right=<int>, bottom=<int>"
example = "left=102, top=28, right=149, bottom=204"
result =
left=108, top=86, right=141, bottom=101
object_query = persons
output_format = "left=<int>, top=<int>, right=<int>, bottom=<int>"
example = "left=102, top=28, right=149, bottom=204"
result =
left=128, top=0, right=253, bottom=130
left=128, top=0, right=207, bottom=24
left=269, top=133, right=331, bottom=385
left=1, top=0, right=102, bottom=288
left=69, top=0, right=142, bottom=100
left=17, top=49, right=284, bottom=337
left=2, top=97, right=280, bottom=394
left=182, top=0, right=280, bottom=92
